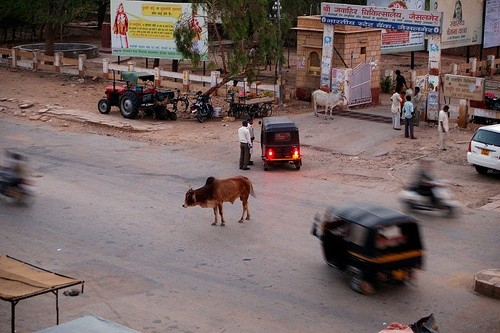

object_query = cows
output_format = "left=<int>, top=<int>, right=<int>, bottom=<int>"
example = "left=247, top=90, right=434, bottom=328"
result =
left=311, top=89, right=344, bottom=119
left=183, top=174, right=256, bottom=226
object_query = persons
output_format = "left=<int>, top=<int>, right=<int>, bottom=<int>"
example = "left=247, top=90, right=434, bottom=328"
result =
left=395, top=69, right=408, bottom=93
left=413, top=86, right=422, bottom=126
left=0, top=152, right=29, bottom=203
left=402, top=95, right=418, bottom=139
left=228, top=80, right=240, bottom=113
left=238, top=120, right=251, bottom=171
left=248, top=118, right=256, bottom=166
left=390, top=88, right=403, bottom=130
left=437, top=105, right=450, bottom=151
left=404, top=157, right=443, bottom=207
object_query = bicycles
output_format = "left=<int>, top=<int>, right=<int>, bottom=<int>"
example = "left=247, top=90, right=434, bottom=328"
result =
left=172, top=88, right=211, bottom=112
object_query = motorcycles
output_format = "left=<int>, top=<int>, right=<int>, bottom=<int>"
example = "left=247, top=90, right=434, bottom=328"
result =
left=190, top=90, right=213, bottom=122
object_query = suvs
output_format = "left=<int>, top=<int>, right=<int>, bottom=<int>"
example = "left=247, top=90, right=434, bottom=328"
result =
left=467, top=122, right=500, bottom=175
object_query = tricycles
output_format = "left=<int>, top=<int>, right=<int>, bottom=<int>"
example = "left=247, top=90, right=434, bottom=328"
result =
left=225, top=92, right=276, bottom=118
left=258, top=117, right=302, bottom=169
left=311, top=203, right=424, bottom=292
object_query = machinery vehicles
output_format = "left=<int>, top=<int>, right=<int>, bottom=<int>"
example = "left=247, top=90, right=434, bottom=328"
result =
left=98, top=68, right=175, bottom=118
